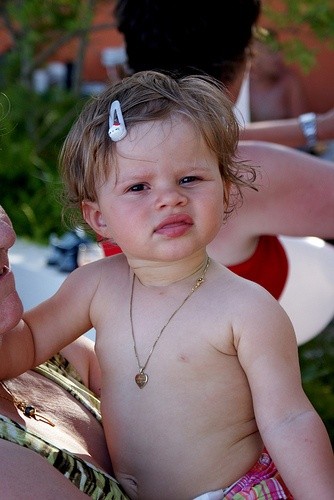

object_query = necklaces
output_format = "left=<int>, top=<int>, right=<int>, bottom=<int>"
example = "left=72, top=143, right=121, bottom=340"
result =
left=0, top=380, right=55, bottom=427
left=129, top=256, right=212, bottom=387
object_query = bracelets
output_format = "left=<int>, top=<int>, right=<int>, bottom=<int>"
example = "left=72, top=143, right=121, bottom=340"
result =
left=298, top=112, right=318, bottom=150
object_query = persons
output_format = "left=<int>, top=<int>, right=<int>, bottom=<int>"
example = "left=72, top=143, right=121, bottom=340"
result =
left=1, top=206, right=136, bottom=500
left=98, top=0, right=333, bottom=299
left=0, top=70, right=333, bottom=498
